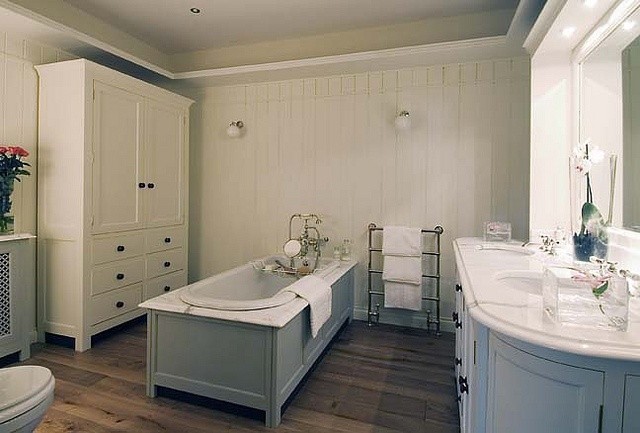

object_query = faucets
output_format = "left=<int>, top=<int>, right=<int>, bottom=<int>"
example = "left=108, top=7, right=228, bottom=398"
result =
left=521, top=235, right=551, bottom=249
left=607, top=261, right=618, bottom=275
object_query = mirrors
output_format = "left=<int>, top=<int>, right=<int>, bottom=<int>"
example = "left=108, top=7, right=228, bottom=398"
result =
left=570, top=2, right=640, bottom=280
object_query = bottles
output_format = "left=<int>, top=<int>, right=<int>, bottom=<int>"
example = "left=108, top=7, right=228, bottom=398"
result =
left=333, top=247, right=340, bottom=262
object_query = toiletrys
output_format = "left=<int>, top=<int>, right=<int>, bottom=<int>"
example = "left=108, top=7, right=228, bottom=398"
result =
left=332, top=246, right=341, bottom=260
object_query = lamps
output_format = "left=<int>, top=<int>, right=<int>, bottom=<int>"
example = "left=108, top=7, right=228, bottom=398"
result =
left=226, top=120, right=247, bottom=138
left=394, top=111, right=412, bottom=133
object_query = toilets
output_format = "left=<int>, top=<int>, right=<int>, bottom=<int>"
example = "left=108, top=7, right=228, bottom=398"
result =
left=0, top=365, right=56, bottom=433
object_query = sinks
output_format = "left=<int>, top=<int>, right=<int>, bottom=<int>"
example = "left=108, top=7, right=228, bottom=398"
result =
left=495, top=270, right=543, bottom=296
left=473, top=243, right=537, bottom=257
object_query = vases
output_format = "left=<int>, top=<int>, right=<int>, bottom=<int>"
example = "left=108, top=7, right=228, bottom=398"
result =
left=0, top=214, right=14, bottom=235
left=572, top=235, right=608, bottom=262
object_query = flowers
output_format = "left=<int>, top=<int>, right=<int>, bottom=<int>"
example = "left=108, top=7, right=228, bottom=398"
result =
left=0, top=145, right=33, bottom=231
left=571, top=139, right=605, bottom=234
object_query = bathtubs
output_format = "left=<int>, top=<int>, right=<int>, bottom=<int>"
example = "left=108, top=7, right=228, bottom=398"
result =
left=137, top=254, right=359, bottom=428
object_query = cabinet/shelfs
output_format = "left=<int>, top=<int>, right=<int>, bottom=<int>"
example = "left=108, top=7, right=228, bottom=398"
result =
left=453, top=266, right=471, bottom=431
left=33, top=59, right=196, bottom=352
left=485, top=329, right=640, bottom=433
left=0, top=234, right=36, bottom=362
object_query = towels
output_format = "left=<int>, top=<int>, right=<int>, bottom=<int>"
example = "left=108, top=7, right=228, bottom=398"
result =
left=271, top=275, right=333, bottom=338
left=381, top=225, right=423, bottom=258
left=382, top=256, right=422, bottom=285
left=384, top=282, right=422, bottom=312
